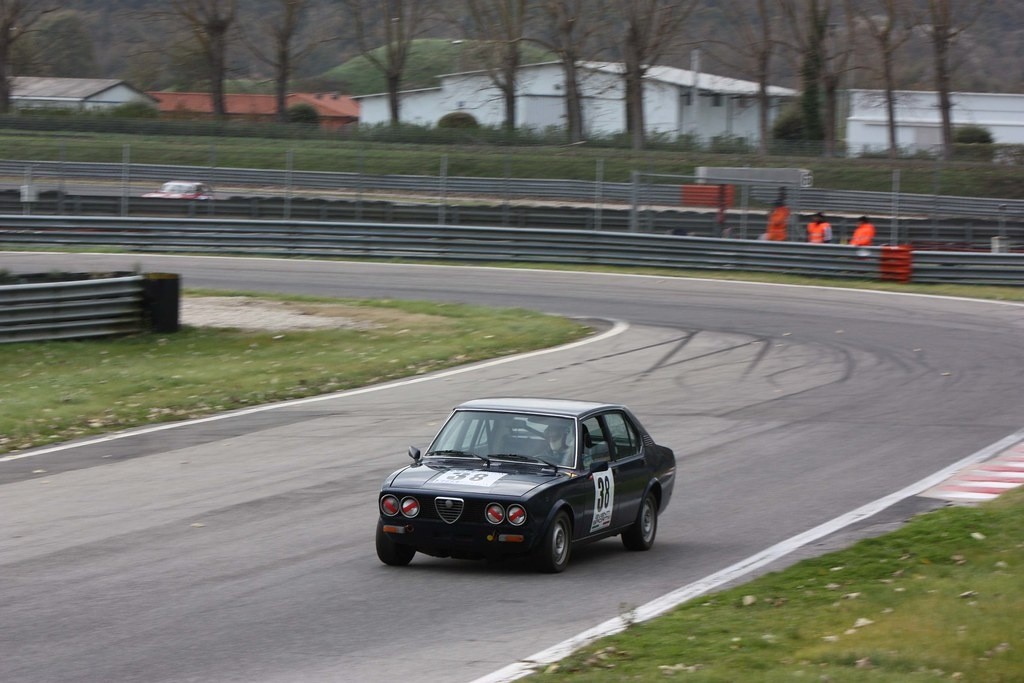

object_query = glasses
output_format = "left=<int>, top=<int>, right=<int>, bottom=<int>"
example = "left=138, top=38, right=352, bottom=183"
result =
left=548, top=433, right=560, bottom=442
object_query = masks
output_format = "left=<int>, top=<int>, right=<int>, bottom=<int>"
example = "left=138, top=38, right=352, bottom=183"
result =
left=550, top=438, right=563, bottom=451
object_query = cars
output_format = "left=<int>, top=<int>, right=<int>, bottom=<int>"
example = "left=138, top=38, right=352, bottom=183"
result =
left=375, top=395, right=678, bottom=574
left=141, top=179, right=214, bottom=200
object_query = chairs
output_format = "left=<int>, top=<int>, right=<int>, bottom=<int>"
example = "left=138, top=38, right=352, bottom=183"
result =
left=484, top=420, right=515, bottom=457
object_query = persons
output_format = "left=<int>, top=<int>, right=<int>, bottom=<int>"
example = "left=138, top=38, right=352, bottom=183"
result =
left=532, top=423, right=592, bottom=467
left=766, top=201, right=790, bottom=240
left=806, top=212, right=831, bottom=244
left=848, top=216, right=876, bottom=245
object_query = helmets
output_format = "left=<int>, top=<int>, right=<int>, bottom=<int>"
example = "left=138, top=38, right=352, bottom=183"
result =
left=543, top=420, right=573, bottom=450
